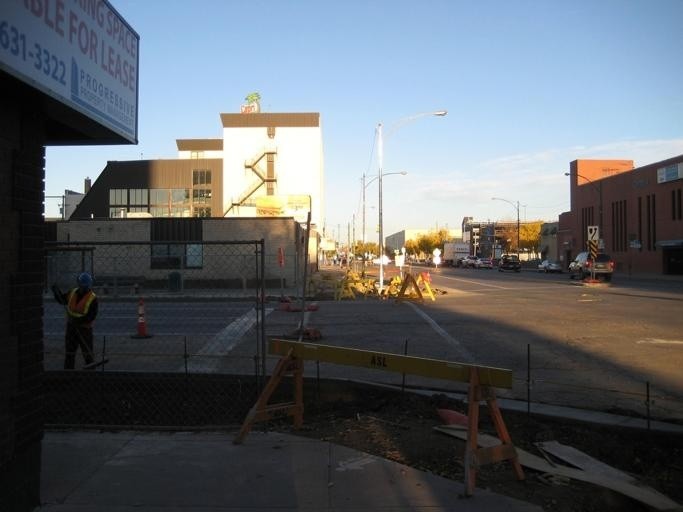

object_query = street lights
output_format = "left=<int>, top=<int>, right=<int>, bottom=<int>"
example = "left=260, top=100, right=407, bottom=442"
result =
left=564, top=172, right=605, bottom=251
left=376, top=109, right=448, bottom=292
left=492, top=193, right=520, bottom=255
left=347, top=169, right=407, bottom=265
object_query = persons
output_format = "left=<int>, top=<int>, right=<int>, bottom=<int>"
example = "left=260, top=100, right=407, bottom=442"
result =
left=51, top=272, right=98, bottom=369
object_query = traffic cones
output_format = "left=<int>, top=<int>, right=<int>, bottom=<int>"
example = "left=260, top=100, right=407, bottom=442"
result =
left=130, top=299, right=153, bottom=340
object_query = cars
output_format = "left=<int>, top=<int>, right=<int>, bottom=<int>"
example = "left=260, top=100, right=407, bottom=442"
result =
left=497, top=255, right=522, bottom=272
left=536, top=258, right=562, bottom=273
left=568, top=251, right=616, bottom=279
left=459, top=256, right=493, bottom=271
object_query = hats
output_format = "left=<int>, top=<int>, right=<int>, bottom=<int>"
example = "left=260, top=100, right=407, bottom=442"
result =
left=78, top=272, right=93, bottom=285
